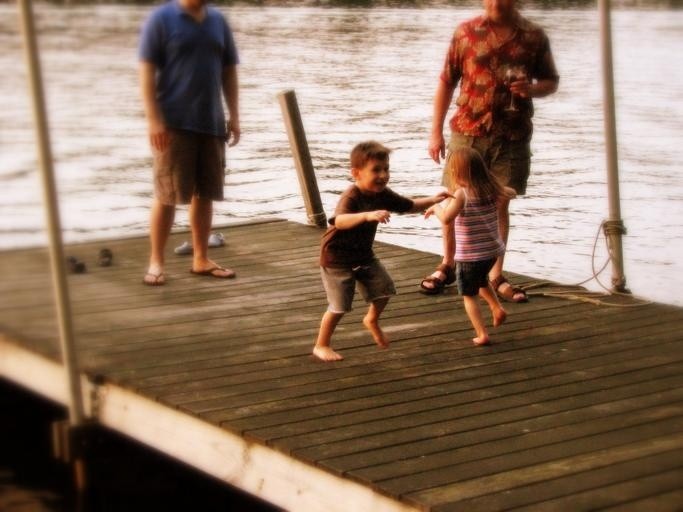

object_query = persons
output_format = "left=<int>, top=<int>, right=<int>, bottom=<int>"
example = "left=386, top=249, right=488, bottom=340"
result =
left=136, top=2, right=241, bottom=285
left=419, top=0, right=560, bottom=303
left=312, top=140, right=457, bottom=363
left=423, top=145, right=517, bottom=346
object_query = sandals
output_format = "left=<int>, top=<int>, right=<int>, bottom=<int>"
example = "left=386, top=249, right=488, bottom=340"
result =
left=487, top=274, right=528, bottom=303
left=418, top=262, right=457, bottom=294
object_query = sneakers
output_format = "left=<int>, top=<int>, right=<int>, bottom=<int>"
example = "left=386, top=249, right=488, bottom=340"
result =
left=208, top=232, right=224, bottom=248
left=98, top=249, right=112, bottom=267
left=174, top=241, right=194, bottom=254
left=65, top=256, right=85, bottom=273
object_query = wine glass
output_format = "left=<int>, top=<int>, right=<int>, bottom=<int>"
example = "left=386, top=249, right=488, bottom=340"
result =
left=503, top=63, right=524, bottom=111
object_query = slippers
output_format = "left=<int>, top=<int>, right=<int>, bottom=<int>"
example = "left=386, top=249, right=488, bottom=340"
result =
left=143, top=273, right=165, bottom=286
left=190, top=265, right=236, bottom=278
